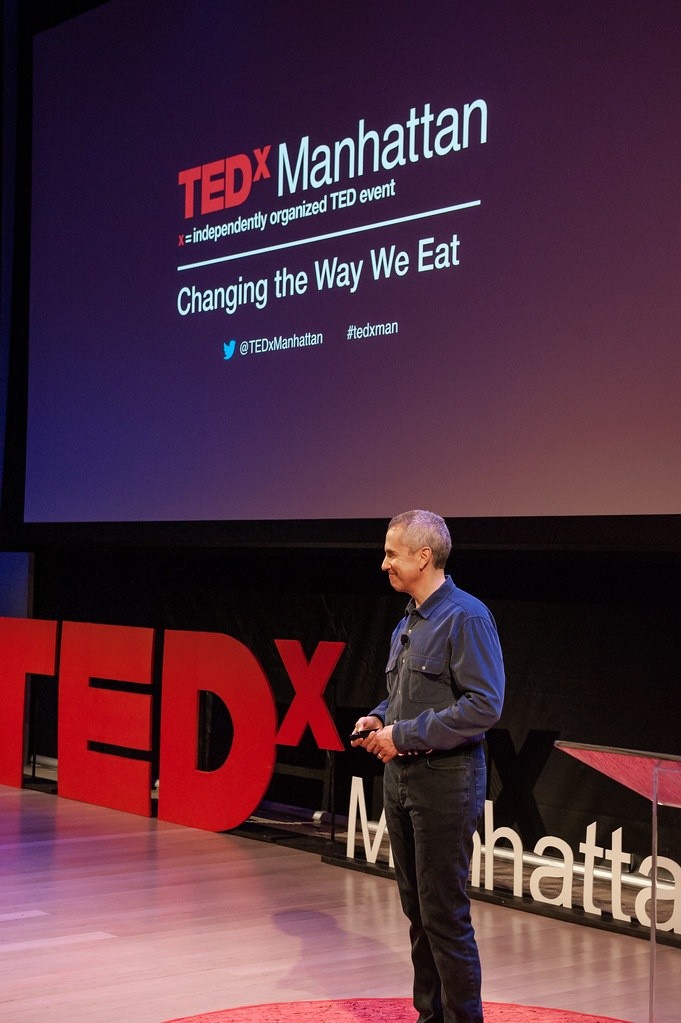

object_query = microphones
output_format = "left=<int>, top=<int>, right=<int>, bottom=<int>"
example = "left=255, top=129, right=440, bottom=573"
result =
left=401, top=634, right=409, bottom=646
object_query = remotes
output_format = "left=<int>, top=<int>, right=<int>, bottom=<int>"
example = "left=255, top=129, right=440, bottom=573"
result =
left=349, top=728, right=378, bottom=740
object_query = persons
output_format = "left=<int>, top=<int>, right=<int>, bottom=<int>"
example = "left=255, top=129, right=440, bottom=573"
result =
left=349, top=511, right=505, bottom=1023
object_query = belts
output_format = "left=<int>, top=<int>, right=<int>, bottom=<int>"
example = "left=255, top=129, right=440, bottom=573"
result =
left=398, top=749, right=433, bottom=756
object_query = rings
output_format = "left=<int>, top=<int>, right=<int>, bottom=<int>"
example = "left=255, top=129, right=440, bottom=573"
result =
left=378, top=753, right=383, bottom=758
